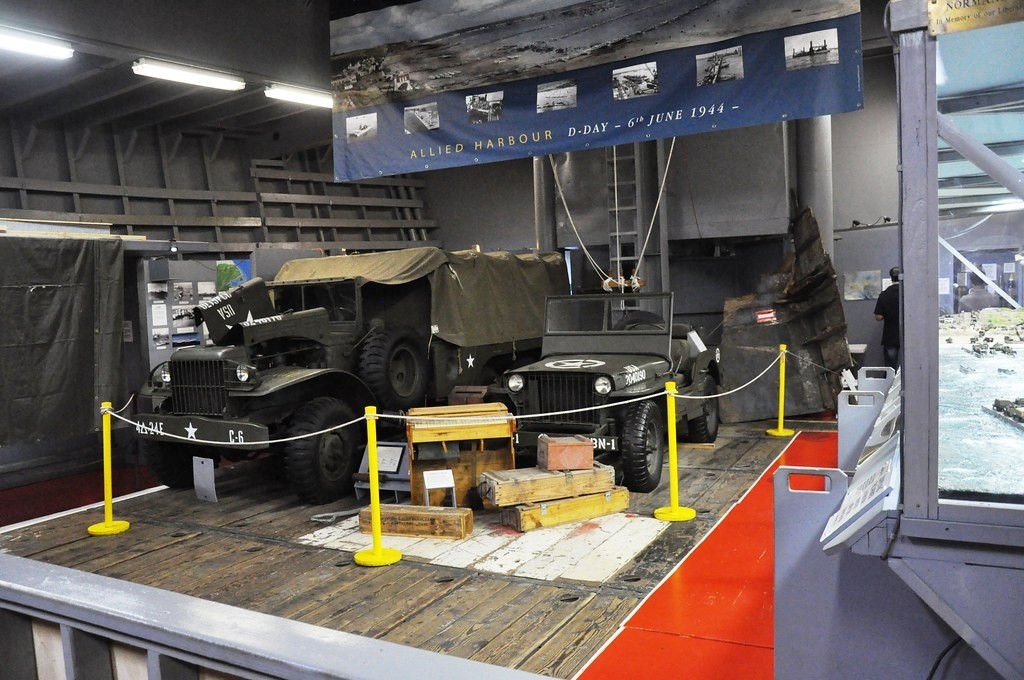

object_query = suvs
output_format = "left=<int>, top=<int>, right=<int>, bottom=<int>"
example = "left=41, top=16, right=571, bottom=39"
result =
left=499, top=289, right=725, bottom=493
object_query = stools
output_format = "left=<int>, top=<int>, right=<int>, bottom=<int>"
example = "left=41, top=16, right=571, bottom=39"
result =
left=421, top=469, right=456, bottom=507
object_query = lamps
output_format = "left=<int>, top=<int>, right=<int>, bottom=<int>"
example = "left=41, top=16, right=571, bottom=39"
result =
left=169, top=239, right=179, bottom=252
left=264, top=84, right=333, bottom=109
left=0, top=27, right=75, bottom=60
left=132, top=58, right=246, bottom=92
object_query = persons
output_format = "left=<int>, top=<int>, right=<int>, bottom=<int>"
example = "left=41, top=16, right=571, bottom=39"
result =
left=957, top=269, right=999, bottom=314
left=872, top=266, right=904, bottom=377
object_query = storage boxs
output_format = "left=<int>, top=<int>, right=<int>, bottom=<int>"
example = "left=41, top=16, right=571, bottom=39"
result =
left=359, top=502, right=474, bottom=541
left=537, top=433, right=594, bottom=469
left=480, top=461, right=615, bottom=507
left=499, top=486, right=631, bottom=534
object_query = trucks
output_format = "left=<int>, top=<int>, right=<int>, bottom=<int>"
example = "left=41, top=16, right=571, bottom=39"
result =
left=130, top=241, right=569, bottom=506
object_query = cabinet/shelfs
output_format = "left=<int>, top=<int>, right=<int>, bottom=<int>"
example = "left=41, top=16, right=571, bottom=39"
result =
left=407, top=402, right=517, bottom=507
